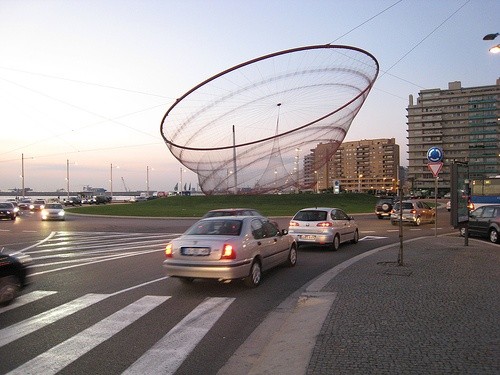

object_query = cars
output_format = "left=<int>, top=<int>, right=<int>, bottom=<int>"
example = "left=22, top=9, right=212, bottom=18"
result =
left=129, top=196, right=159, bottom=202
left=162, top=215, right=298, bottom=287
left=404, top=192, right=442, bottom=199
left=42, top=203, right=66, bottom=221
left=288, top=207, right=359, bottom=250
left=390, top=200, right=436, bottom=226
left=5, top=198, right=46, bottom=216
left=0, top=246, right=33, bottom=304
left=47, top=194, right=112, bottom=206
left=191, top=208, right=279, bottom=234
left=459, top=205, right=500, bottom=244
left=444, top=193, right=451, bottom=199
left=0, top=202, right=16, bottom=220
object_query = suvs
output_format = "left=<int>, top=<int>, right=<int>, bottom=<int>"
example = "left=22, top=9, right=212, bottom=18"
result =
left=375, top=196, right=400, bottom=219
